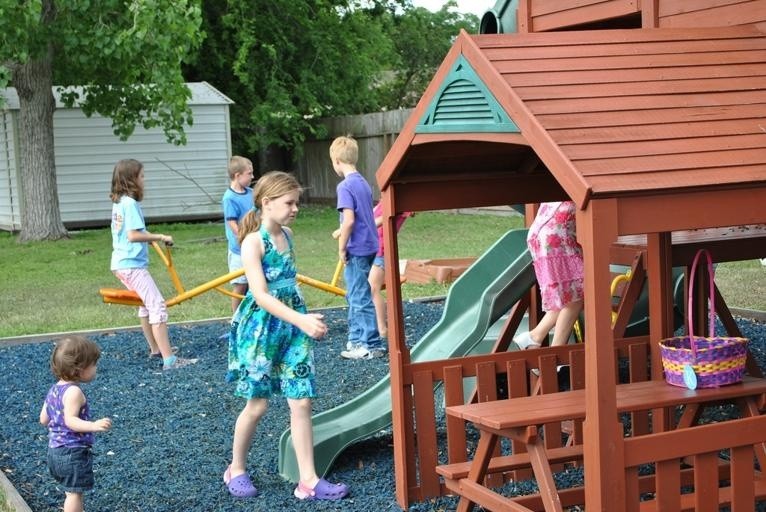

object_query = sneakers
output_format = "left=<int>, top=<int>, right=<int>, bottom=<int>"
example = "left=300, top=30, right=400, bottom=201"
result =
left=163, top=357, right=197, bottom=371
left=340, top=341, right=384, bottom=361
left=512, top=331, right=542, bottom=377
left=150, top=347, right=179, bottom=359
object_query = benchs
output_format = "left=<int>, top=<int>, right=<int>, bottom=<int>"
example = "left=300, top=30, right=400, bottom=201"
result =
left=434, top=413, right=766, bottom=512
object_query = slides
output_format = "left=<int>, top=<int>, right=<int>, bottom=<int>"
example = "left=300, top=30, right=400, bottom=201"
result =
left=277, top=227, right=536, bottom=483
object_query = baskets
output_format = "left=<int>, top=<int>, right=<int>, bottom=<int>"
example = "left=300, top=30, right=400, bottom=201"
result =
left=658, top=248, right=750, bottom=389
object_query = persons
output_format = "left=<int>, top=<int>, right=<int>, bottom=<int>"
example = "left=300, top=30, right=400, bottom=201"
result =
left=223, top=155, right=256, bottom=312
left=509, top=202, right=585, bottom=378
left=367, top=198, right=417, bottom=340
left=38, top=334, right=112, bottom=512
left=222, top=169, right=348, bottom=502
left=329, top=135, right=385, bottom=360
left=109, top=158, right=200, bottom=371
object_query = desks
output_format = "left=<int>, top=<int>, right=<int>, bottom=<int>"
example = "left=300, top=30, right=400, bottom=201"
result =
left=443, top=377, right=766, bottom=512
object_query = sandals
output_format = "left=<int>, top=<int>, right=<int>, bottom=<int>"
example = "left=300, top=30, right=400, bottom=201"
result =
left=223, top=464, right=257, bottom=498
left=294, top=477, right=349, bottom=501
